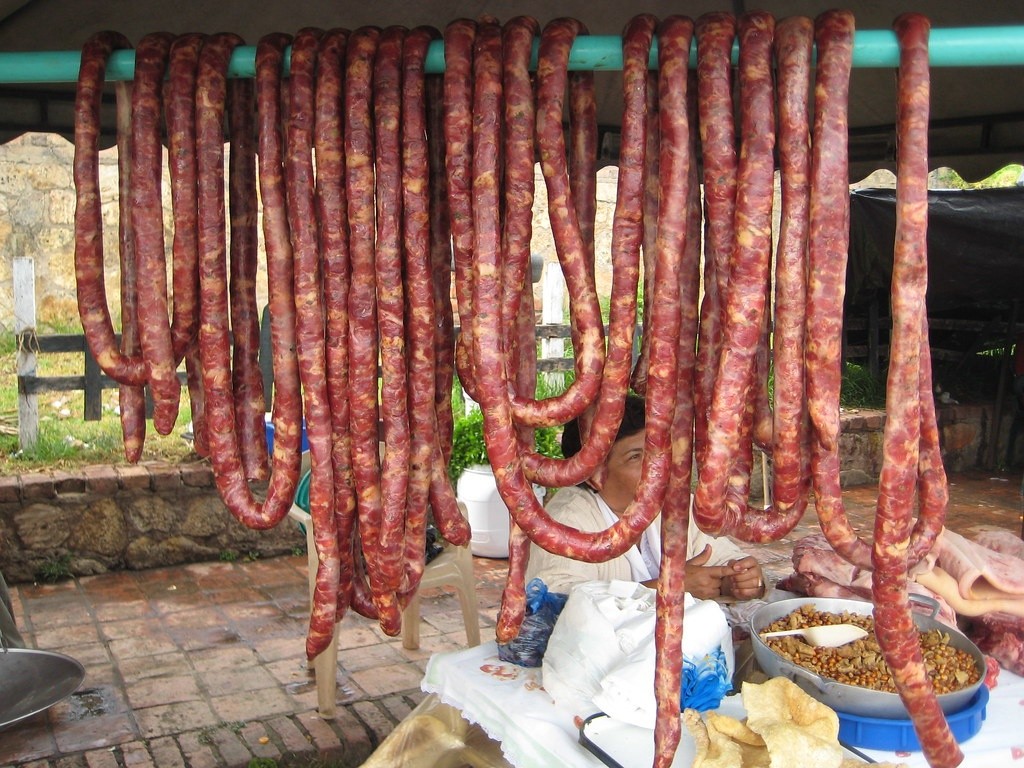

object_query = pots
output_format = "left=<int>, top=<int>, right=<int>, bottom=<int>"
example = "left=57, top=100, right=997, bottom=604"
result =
left=750, top=593, right=986, bottom=718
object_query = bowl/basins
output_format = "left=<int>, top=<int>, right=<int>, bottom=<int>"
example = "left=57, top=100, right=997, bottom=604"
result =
left=0, top=647, right=85, bottom=731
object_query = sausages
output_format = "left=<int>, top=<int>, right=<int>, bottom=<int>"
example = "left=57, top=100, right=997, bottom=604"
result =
left=74, top=8, right=965, bottom=768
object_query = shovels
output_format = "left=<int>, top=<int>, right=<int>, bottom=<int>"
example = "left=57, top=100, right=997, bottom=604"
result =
left=758, top=624, right=869, bottom=648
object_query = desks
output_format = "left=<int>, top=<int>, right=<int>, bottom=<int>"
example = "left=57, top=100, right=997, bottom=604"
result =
left=423, top=549, right=1024, bottom=767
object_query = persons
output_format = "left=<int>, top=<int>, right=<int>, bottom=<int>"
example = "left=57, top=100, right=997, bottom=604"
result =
left=998, top=332, right=1024, bottom=473
left=525, top=393, right=767, bottom=601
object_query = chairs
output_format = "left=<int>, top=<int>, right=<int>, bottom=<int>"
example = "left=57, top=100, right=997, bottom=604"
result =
left=270, top=442, right=493, bottom=718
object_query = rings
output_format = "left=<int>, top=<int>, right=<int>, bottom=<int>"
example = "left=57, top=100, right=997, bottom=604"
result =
left=759, top=579, right=763, bottom=587
